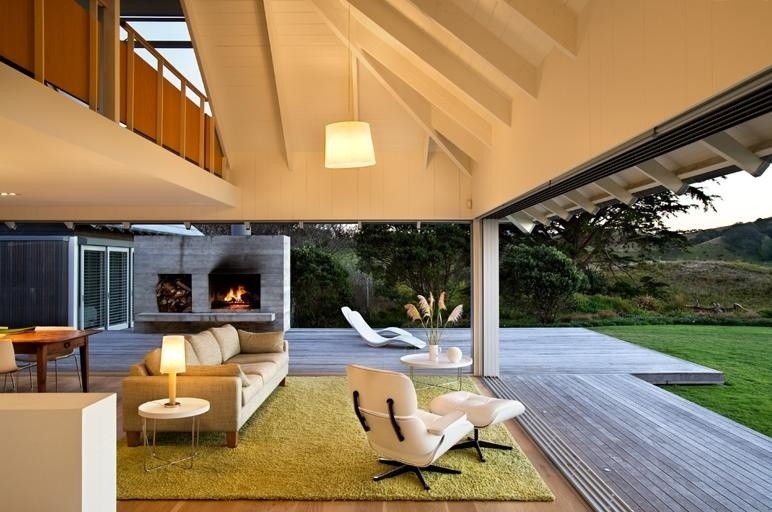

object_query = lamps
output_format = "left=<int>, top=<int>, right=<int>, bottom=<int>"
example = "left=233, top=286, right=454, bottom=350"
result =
left=324, top=0, right=377, bottom=169
left=160, top=336, right=186, bottom=407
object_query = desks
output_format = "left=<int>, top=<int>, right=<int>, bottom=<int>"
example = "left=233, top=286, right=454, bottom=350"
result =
left=0, top=326, right=105, bottom=393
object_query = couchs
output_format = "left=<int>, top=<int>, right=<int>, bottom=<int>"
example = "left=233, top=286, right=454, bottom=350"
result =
left=121, top=339, right=289, bottom=448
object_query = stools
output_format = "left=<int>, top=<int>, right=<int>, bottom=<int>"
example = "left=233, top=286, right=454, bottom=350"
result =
left=430, top=391, right=525, bottom=462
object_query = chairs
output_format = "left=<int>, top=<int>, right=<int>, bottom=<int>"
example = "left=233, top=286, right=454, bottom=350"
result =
left=0, top=339, right=33, bottom=393
left=341, top=306, right=412, bottom=336
left=347, top=363, right=475, bottom=490
left=348, top=311, right=426, bottom=350
left=15, top=326, right=81, bottom=392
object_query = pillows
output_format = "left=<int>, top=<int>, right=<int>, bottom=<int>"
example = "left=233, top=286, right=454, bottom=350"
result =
left=209, top=323, right=240, bottom=362
left=237, top=329, right=285, bottom=353
left=167, top=335, right=199, bottom=365
left=139, top=344, right=178, bottom=376
left=186, top=331, right=222, bottom=365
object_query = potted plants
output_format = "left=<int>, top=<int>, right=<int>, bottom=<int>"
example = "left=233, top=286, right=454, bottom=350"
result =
left=404, top=292, right=464, bottom=362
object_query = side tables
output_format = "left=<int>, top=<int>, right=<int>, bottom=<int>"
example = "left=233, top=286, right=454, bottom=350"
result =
left=138, top=397, right=211, bottom=470
left=399, top=353, right=473, bottom=401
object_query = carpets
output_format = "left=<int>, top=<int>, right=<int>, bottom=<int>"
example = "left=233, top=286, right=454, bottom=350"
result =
left=104, top=376, right=554, bottom=501
